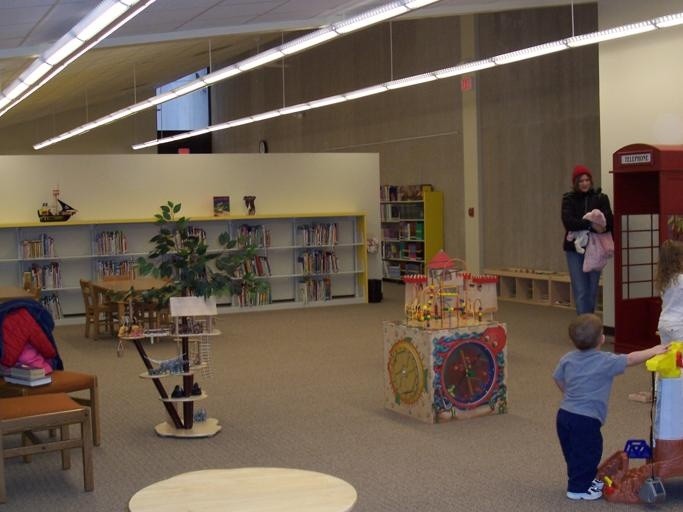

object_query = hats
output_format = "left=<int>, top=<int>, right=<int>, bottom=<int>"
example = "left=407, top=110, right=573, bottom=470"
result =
left=572, top=164, right=593, bottom=187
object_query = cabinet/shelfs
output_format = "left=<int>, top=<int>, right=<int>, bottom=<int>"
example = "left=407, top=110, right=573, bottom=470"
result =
left=120, top=328, right=223, bottom=439
left=483, top=267, right=604, bottom=318
left=0, top=212, right=368, bottom=326
left=380, top=183, right=443, bottom=280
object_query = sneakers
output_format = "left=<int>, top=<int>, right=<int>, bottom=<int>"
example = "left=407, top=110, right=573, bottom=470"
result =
left=592, top=478, right=605, bottom=489
left=566, top=485, right=603, bottom=500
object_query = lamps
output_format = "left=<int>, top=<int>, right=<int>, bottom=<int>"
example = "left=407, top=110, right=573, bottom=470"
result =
left=31, top=1, right=437, bottom=151
left=130, top=0, right=683, bottom=150
left=0, top=1, right=155, bottom=119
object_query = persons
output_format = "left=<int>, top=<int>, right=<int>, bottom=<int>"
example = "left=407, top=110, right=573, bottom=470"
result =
left=561, top=165, right=614, bottom=315
left=551, top=313, right=672, bottom=500
left=655, top=240, right=683, bottom=479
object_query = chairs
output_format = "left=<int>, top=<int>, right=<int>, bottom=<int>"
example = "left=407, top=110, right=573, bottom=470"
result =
left=23, top=272, right=41, bottom=303
left=0, top=296, right=100, bottom=463
left=0, top=392, right=94, bottom=493
left=79, top=279, right=117, bottom=341
left=103, top=276, right=130, bottom=282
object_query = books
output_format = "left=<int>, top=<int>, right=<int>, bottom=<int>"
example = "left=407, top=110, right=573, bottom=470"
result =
left=164, top=256, right=207, bottom=281
left=94, top=230, right=135, bottom=281
left=380, top=184, right=434, bottom=201
left=296, top=223, right=339, bottom=301
left=382, top=223, right=424, bottom=240
left=382, top=242, right=424, bottom=261
left=383, top=261, right=423, bottom=280
left=213, top=196, right=230, bottom=219
left=228, top=223, right=273, bottom=306
left=4, top=366, right=52, bottom=387
left=20, top=234, right=63, bottom=321
left=380, top=203, right=400, bottom=222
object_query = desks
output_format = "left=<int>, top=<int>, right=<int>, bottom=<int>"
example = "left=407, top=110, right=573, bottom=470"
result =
left=0, top=285, right=35, bottom=303
left=92, top=280, right=169, bottom=294
left=128, top=467, right=357, bottom=512
